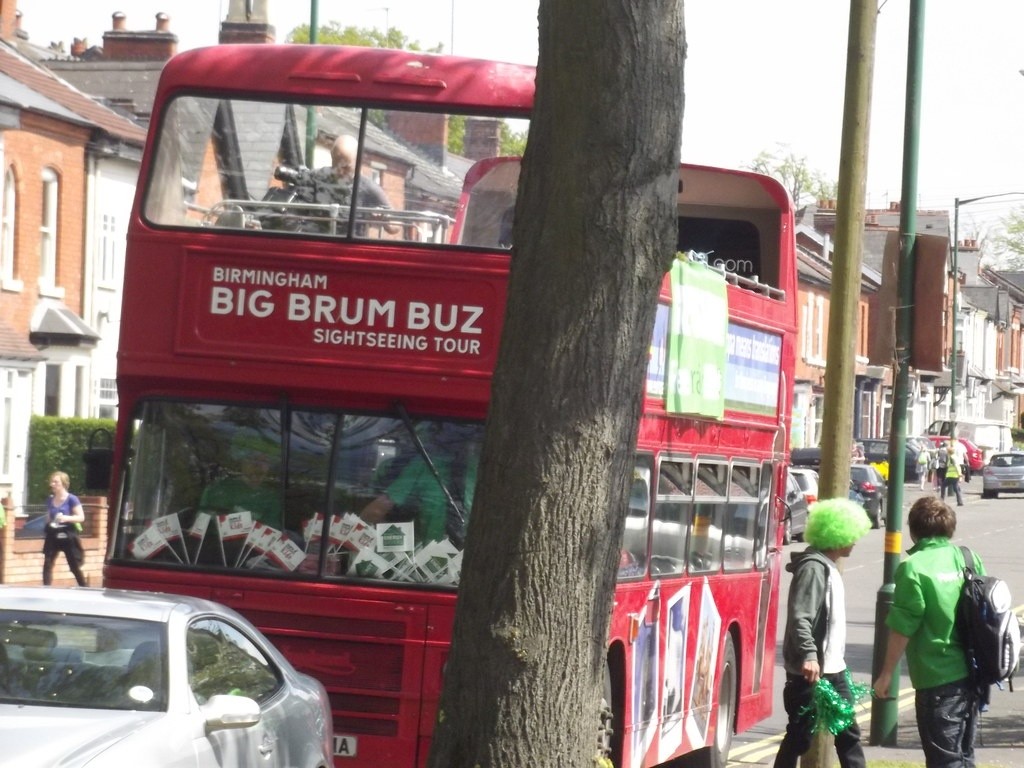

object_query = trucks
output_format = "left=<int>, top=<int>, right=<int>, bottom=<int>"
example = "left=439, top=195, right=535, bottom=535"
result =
left=920, top=419, right=1014, bottom=465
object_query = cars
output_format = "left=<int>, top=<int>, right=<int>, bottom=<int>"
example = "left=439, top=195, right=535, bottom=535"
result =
left=850, top=465, right=888, bottom=529
left=783, top=473, right=807, bottom=545
left=905, top=436, right=935, bottom=451
left=928, top=436, right=985, bottom=474
left=983, top=452, right=1024, bottom=499
left=788, top=469, right=819, bottom=503
left=0, top=585, right=335, bottom=768
left=855, top=438, right=921, bottom=481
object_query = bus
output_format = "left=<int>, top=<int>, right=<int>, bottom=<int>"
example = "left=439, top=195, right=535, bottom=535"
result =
left=95, top=44, right=801, bottom=768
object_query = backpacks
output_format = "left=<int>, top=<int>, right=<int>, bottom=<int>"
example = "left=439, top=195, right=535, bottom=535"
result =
left=918, top=452, right=927, bottom=465
left=957, top=545, right=1020, bottom=686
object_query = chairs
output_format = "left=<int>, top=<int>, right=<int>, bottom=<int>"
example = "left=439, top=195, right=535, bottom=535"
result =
left=0, top=628, right=161, bottom=712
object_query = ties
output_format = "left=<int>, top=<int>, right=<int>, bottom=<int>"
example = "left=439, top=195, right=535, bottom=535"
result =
left=444, top=442, right=466, bottom=552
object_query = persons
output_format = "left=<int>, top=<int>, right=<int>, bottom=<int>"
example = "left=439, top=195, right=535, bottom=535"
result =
left=874, top=496, right=988, bottom=768
left=104, top=641, right=162, bottom=703
left=851, top=439, right=865, bottom=464
left=198, top=417, right=482, bottom=579
left=915, top=437, right=970, bottom=505
left=773, top=498, right=866, bottom=768
left=43, top=472, right=89, bottom=586
left=279, top=134, right=401, bottom=239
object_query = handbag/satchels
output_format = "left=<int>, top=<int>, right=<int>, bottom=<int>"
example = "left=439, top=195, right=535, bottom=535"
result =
left=944, top=464, right=959, bottom=481
left=933, top=449, right=939, bottom=469
